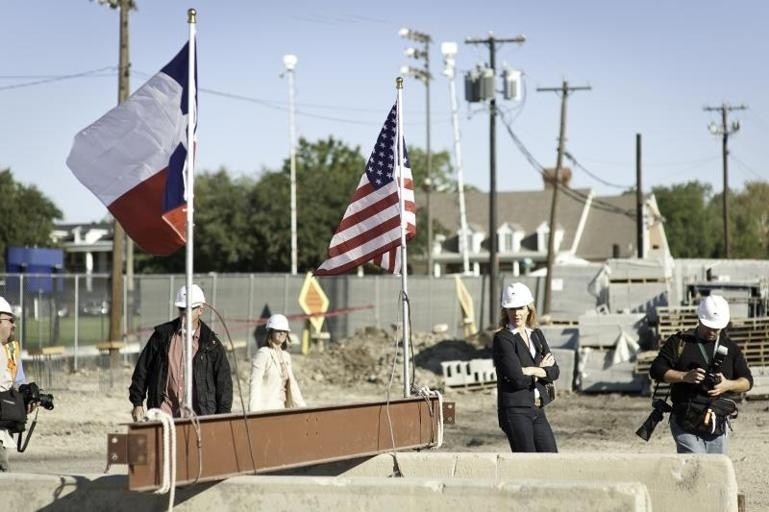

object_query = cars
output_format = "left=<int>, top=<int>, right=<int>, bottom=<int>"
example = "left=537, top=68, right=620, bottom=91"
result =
left=3, top=297, right=143, bottom=323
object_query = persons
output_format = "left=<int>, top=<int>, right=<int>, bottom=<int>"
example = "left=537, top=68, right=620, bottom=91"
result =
left=248, top=314, right=307, bottom=412
left=0, top=296, right=41, bottom=472
left=650, top=294, right=753, bottom=452
left=493, top=282, right=561, bottom=452
left=128, top=285, right=233, bottom=421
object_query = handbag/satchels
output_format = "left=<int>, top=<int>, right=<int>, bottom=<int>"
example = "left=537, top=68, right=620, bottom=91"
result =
left=536, top=329, right=556, bottom=408
left=675, top=385, right=742, bottom=441
left=1, top=386, right=28, bottom=434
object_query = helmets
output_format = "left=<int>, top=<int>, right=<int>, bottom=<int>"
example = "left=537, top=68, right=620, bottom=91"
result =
left=500, top=281, right=537, bottom=311
left=264, top=313, right=291, bottom=334
left=174, top=283, right=207, bottom=308
left=0, top=295, right=15, bottom=316
left=696, top=294, right=732, bottom=330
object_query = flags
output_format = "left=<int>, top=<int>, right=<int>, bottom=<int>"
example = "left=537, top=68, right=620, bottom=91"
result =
left=66, top=38, right=198, bottom=255
left=313, top=90, right=415, bottom=277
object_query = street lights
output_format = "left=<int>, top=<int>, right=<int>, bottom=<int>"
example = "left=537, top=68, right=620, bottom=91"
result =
left=278, top=50, right=304, bottom=272
left=395, top=23, right=440, bottom=279
left=701, top=103, right=753, bottom=258
left=440, top=37, right=477, bottom=275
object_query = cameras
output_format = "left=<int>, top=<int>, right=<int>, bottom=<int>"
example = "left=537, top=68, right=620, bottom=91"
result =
left=19, top=382, right=53, bottom=410
left=701, top=345, right=727, bottom=392
left=635, top=399, right=671, bottom=441
